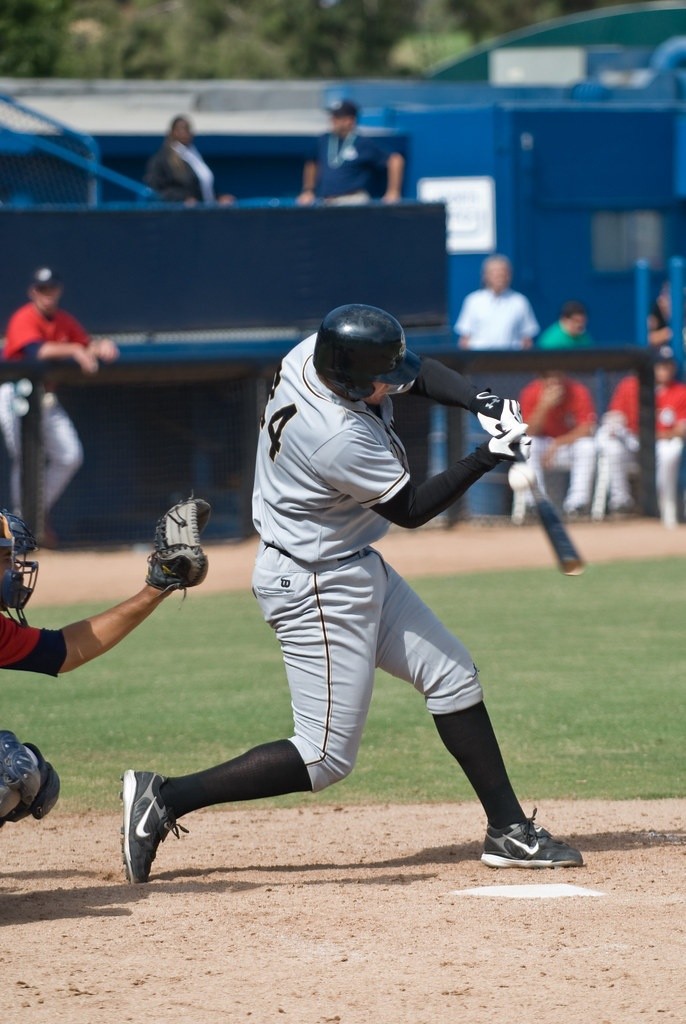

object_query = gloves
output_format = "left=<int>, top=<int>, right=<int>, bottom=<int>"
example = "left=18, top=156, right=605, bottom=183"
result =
left=469, top=391, right=523, bottom=436
left=487, top=425, right=532, bottom=462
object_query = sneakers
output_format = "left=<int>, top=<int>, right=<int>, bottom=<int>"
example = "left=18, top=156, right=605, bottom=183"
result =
left=481, top=808, right=584, bottom=867
left=119, top=769, right=190, bottom=884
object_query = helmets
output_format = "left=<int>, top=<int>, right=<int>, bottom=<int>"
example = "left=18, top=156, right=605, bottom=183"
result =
left=30, top=265, right=65, bottom=289
left=0, top=508, right=40, bottom=628
left=314, top=303, right=423, bottom=401
left=654, top=345, right=678, bottom=363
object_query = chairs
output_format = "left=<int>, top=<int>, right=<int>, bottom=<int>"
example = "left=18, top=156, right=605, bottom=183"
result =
left=597, top=437, right=683, bottom=523
left=511, top=438, right=603, bottom=524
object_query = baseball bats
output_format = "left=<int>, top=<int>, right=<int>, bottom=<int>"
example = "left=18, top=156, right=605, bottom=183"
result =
left=505, top=436, right=586, bottom=579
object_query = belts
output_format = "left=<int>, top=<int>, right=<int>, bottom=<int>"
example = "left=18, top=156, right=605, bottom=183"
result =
left=263, top=541, right=360, bottom=561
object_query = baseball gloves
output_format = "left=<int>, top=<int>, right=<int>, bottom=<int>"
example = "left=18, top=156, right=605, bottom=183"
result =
left=144, top=483, right=213, bottom=604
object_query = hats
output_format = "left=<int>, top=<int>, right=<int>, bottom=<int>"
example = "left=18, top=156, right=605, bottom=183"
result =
left=324, top=99, right=356, bottom=115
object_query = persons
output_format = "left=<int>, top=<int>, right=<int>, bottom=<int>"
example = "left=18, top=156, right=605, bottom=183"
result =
left=0, top=499, right=212, bottom=830
left=0, top=265, right=119, bottom=552
left=295, top=102, right=408, bottom=204
left=453, top=253, right=686, bottom=527
left=147, top=116, right=233, bottom=206
left=118, top=304, right=583, bottom=891
